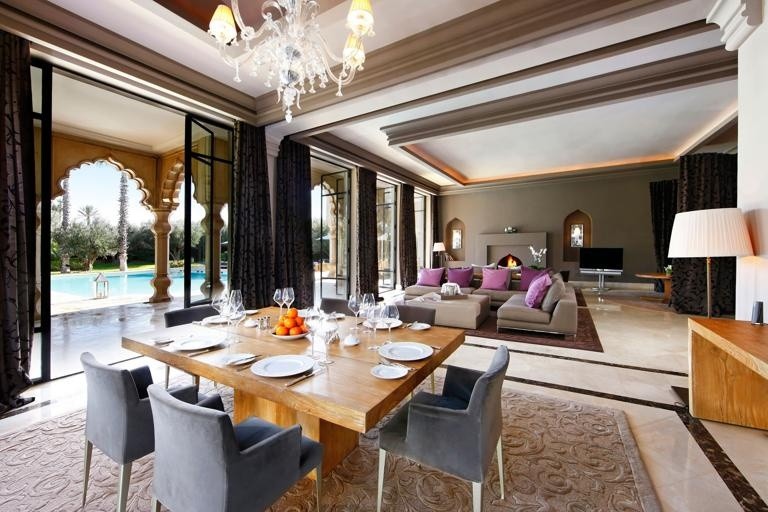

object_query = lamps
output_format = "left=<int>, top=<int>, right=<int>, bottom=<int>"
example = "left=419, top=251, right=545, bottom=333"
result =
left=208, top=0, right=376, bottom=124
left=433, top=242, right=445, bottom=256
left=667, top=208, right=753, bottom=317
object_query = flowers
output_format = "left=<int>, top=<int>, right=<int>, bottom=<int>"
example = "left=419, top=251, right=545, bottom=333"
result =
left=529, top=245, right=547, bottom=265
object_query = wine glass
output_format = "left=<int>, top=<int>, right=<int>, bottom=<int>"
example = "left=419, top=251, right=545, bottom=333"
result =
left=281, top=286, right=297, bottom=310
left=296, top=293, right=404, bottom=365
left=273, top=288, right=286, bottom=313
left=212, top=288, right=245, bottom=345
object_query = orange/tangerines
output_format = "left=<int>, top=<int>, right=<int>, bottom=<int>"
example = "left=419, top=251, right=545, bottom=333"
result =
left=274, top=307, right=307, bottom=335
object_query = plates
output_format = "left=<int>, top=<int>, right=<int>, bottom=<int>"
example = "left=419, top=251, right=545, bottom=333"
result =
left=201, top=313, right=230, bottom=324
left=244, top=310, right=258, bottom=314
left=370, top=364, right=408, bottom=381
left=175, top=334, right=226, bottom=351
left=155, top=337, right=174, bottom=344
left=221, top=352, right=255, bottom=367
left=378, top=341, right=433, bottom=362
left=269, top=330, right=309, bottom=342
left=409, top=323, right=430, bottom=332
left=249, top=353, right=314, bottom=379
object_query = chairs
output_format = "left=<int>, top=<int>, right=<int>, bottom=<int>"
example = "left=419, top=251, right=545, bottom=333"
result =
left=319, top=299, right=361, bottom=317
left=161, top=304, right=222, bottom=392
left=80, top=350, right=212, bottom=511
left=144, top=381, right=325, bottom=511
left=388, top=303, right=436, bottom=326
left=372, top=342, right=509, bottom=510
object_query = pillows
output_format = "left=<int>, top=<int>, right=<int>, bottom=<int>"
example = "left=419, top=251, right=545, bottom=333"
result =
left=417, top=266, right=565, bottom=312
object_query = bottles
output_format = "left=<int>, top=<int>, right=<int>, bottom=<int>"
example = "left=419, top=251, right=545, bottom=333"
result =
left=256, top=316, right=271, bottom=329
left=751, top=301, right=764, bottom=324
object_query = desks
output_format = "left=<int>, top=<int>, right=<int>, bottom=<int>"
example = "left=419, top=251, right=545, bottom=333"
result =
left=688, top=318, right=768, bottom=430
left=120, top=304, right=469, bottom=482
left=635, top=273, right=672, bottom=303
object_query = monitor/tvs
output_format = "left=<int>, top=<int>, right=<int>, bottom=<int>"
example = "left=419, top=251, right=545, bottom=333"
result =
left=579, top=248, right=623, bottom=271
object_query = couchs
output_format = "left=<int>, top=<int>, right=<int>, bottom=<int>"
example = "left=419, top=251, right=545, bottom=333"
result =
left=404, top=278, right=578, bottom=341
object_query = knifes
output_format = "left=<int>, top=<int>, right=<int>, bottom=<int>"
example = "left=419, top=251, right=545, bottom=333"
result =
left=185, top=345, right=227, bottom=358
left=285, top=366, right=327, bottom=388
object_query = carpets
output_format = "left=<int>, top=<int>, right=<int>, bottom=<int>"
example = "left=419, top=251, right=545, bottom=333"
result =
left=604, top=290, right=675, bottom=314
left=575, top=288, right=587, bottom=306
left=464, top=308, right=604, bottom=352
left=0, top=375, right=663, bottom=511
